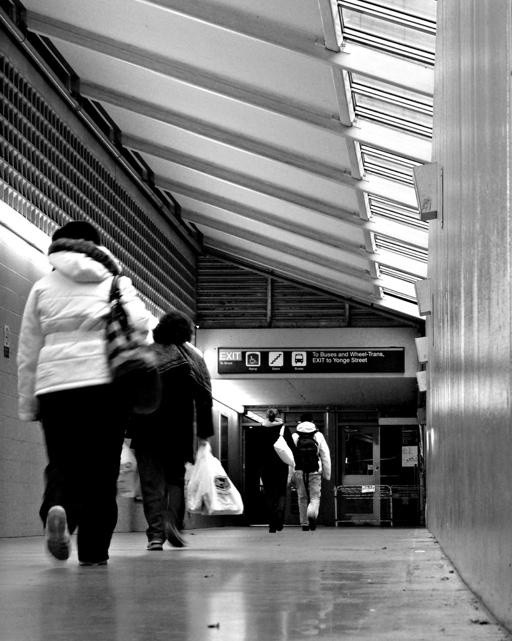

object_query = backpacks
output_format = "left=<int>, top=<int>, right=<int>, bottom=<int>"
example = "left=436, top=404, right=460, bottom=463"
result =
left=294, top=430, right=319, bottom=473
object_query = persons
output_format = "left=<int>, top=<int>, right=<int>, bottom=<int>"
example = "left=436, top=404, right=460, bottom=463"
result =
left=257, top=408, right=300, bottom=532
left=15, top=220, right=151, bottom=566
left=123, top=310, right=214, bottom=550
left=287, top=413, right=331, bottom=530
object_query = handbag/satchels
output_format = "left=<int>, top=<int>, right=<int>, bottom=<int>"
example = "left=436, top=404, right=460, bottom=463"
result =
left=273, top=423, right=296, bottom=468
left=101, top=274, right=162, bottom=415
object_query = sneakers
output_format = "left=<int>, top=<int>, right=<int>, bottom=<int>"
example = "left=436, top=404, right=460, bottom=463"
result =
left=164, top=521, right=186, bottom=547
left=308, top=516, right=316, bottom=530
left=146, top=540, right=163, bottom=550
left=45, top=505, right=72, bottom=560
